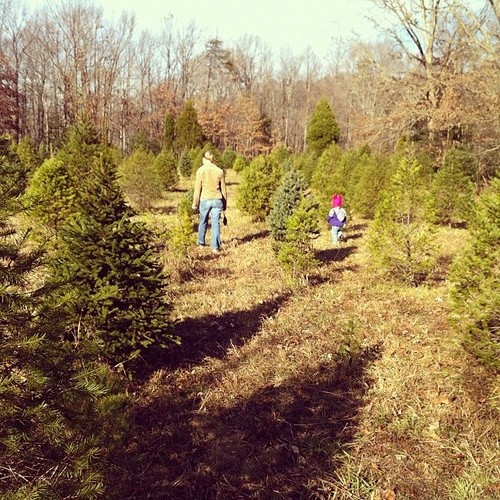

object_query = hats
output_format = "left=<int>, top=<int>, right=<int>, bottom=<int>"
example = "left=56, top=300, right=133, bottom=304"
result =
left=333, top=194, right=343, bottom=207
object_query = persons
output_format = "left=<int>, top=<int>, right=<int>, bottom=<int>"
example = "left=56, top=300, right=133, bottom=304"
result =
left=192, top=151, right=227, bottom=253
left=327, top=196, right=347, bottom=245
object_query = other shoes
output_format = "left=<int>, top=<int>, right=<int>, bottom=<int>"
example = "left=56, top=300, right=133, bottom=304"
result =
left=340, top=236, right=344, bottom=241
